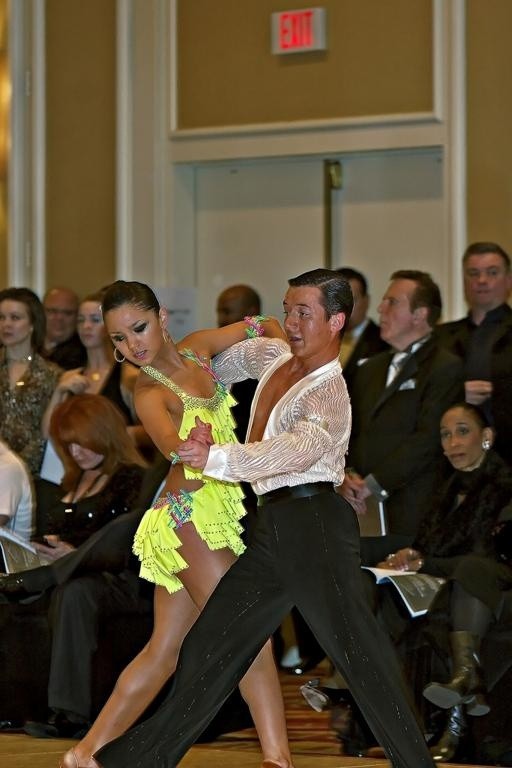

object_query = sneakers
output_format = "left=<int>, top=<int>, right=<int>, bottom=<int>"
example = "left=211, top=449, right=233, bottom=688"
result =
left=25, top=715, right=83, bottom=739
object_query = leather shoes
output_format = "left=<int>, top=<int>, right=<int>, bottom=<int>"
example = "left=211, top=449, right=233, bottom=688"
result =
left=300, top=678, right=353, bottom=712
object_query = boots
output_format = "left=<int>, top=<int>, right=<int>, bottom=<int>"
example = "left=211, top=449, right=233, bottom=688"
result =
left=423, top=630, right=491, bottom=716
left=429, top=704, right=467, bottom=764
